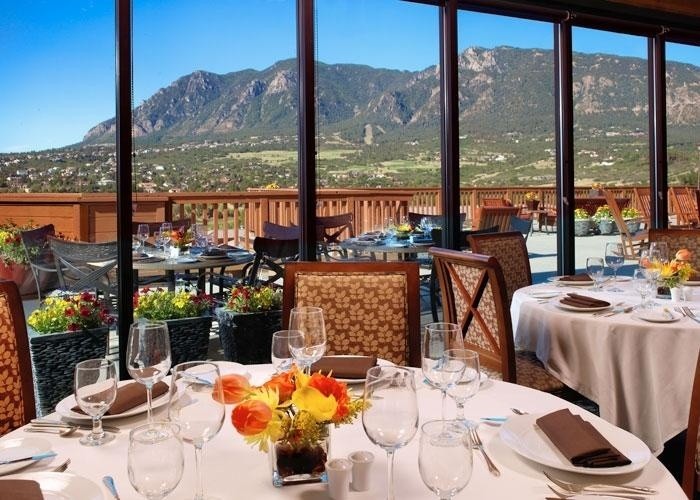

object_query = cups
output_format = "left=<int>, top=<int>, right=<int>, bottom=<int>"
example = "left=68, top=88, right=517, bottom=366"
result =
left=126, top=422, right=184, bottom=498
left=325, top=457, right=353, bottom=498
left=348, top=450, right=376, bottom=492
left=271, top=331, right=306, bottom=376
left=418, top=420, right=474, bottom=498
left=288, top=307, right=327, bottom=374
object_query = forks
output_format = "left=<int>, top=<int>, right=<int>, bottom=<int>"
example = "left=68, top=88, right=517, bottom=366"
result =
left=546, top=483, right=645, bottom=500
left=542, top=470, right=656, bottom=493
left=469, top=429, right=500, bottom=477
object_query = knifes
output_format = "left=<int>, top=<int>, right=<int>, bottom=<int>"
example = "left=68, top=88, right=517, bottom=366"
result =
left=31, top=419, right=121, bottom=432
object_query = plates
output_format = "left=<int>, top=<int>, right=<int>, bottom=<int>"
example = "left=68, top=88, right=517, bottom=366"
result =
left=423, top=360, right=478, bottom=382
left=0, top=471, right=105, bottom=498
left=132, top=244, right=250, bottom=263
left=55, top=378, right=185, bottom=422
left=529, top=275, right=700, bottom=323
left=499, top=413, right=651, bottom=475
left=311, top=354, right=397, bottom=385
left=354, top=234, right=436, bottom=248
left=186, top=363, right=277, bottom=388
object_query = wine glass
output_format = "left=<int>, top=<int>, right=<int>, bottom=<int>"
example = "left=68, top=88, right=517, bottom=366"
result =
left=587, top=241, right=669, bottom=312
left=167, top=361, right=226, bottom=499
left=124, top=320, right=172, bottom=445
left=423, top=322, right=466, bottom=434
left=132, top=223, right=213, bottom=263
left=361, top=365, right=420, bottom=499
left=74, top=358, right=118, bottom=447
left=371, top=216, right=432, bottom=240
left=440, top=348, right=482, bottom=433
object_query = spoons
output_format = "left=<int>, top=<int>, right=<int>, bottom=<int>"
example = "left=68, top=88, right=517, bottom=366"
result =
left=22, top=426, right=80, bottom=438
